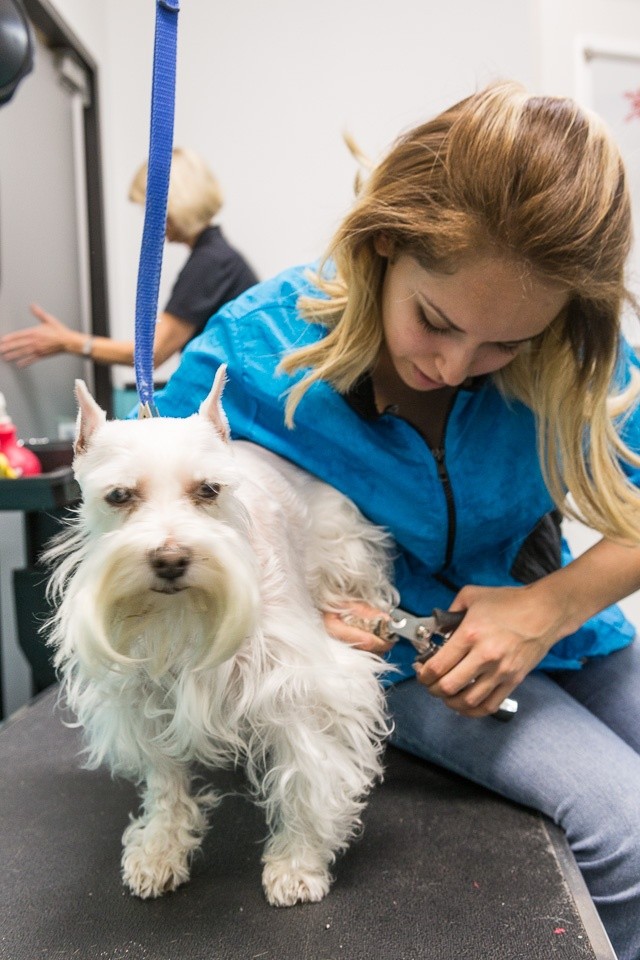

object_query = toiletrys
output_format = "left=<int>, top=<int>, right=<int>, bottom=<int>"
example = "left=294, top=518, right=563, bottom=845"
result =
left=1, top=395, right=43, bottom=479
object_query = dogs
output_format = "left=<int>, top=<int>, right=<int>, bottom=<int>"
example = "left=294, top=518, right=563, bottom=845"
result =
left=33, top=361, right=397, bottom=907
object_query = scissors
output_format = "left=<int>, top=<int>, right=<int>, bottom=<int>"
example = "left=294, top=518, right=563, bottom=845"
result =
left=365, top=607, right=518, bottom=721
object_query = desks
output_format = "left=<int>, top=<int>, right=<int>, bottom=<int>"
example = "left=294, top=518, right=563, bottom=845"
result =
left=0, top=673, right=628, bottom=960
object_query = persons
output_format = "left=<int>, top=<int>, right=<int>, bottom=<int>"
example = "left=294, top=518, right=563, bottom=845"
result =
left=1, top=146, right=260, bottom=371
left=133, top=80, right=640, bottom=960
